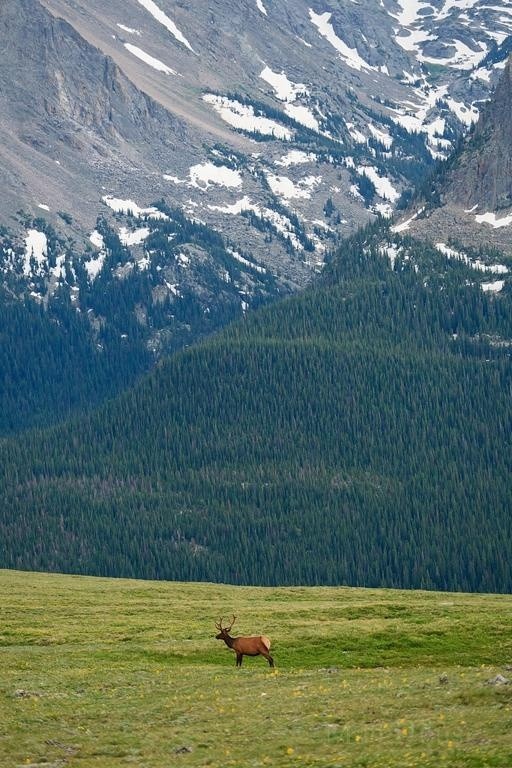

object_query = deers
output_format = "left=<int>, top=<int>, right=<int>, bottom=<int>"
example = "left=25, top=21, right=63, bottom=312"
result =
left=214, top=613, right=275, bottom=668
